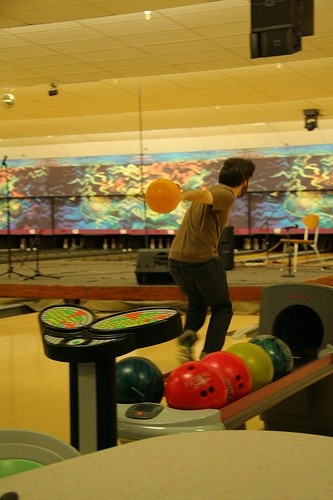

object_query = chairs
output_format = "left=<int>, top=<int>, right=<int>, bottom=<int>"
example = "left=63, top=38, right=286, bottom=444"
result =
left=279, top=214, right=324, bottom=273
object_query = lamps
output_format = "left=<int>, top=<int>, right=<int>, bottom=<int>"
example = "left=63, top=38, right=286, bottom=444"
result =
left=48, top=83, right=59, bottom=95
left=302, top=109, right=319, bottom=132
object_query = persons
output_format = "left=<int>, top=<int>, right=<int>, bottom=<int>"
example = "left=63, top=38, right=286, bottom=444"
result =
left=169, top=158, right=255, bottom=365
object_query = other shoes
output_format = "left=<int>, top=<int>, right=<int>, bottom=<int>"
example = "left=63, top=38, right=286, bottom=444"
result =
left=178, top=342, right=191, bottom=363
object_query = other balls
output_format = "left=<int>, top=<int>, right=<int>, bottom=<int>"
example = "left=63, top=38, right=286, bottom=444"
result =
left=165, top=333, right=295, bottom=412
left=111, top=356, right=163, bottom=403
left=147, top=179, right=181, bottom=215
